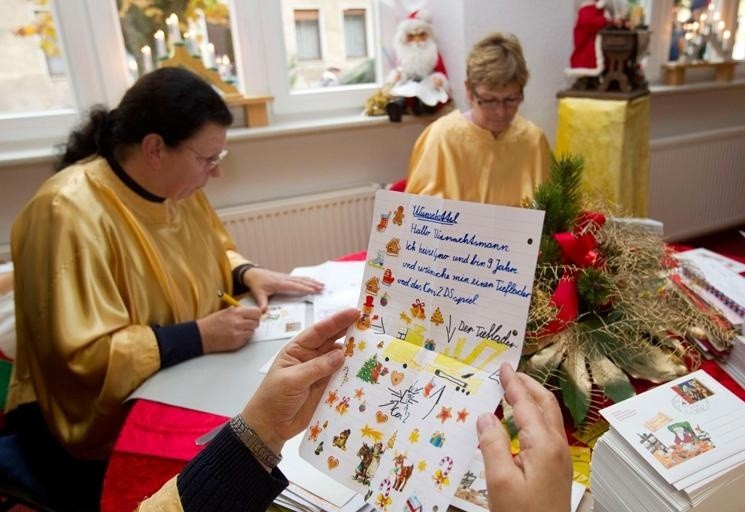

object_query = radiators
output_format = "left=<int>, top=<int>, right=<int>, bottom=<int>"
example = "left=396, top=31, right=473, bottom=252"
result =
left=214, top=183, right=384, bottom=274
left=648, top=125, right=745, bottom=244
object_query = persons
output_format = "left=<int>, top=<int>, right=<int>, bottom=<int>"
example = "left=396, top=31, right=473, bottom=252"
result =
left=2, top=67, right=326, bottom=505
left=403, top=33, right=556, bottom=209
left=383, top=4, right=452, bottom=124
left=133, top=307, right=574, bottom=512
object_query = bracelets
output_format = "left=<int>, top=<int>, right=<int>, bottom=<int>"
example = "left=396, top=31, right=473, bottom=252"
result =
left=226, top=414, right=283, bottom=470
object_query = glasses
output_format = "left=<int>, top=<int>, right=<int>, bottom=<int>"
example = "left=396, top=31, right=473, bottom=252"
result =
left=179, top=141, right=228, bottom=171
left=471, top=86, right=524, bottom=110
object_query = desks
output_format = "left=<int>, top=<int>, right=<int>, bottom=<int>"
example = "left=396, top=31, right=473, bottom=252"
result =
left=103, top=232, right=744, bottom=512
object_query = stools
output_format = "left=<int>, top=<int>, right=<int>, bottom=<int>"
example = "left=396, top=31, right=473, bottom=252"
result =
left=1, top=399, right=105, bottom=512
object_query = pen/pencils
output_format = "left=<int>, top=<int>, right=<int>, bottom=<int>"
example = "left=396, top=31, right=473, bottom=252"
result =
left=217, top=290, right=241, bottom=307
